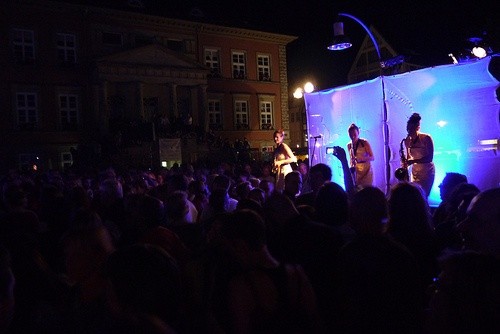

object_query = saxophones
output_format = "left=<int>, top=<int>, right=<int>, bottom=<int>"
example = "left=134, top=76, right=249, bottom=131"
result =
left=395, top=138, right=410, bottom=182
left=349, top=144, right=356, bottom=173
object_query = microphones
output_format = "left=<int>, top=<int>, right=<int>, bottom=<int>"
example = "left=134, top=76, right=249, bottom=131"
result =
left=310, top=134, right=323, bottom=138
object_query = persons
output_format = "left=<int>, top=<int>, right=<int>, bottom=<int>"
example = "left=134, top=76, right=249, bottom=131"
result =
left=271, top=131, right=297, bottom=179
left=347, top=125, right=372, bottom=190
left=108, top=111, right=251, bottom=146
left=0, top=146, right=500, bottom=334
left=399, top=113, right=434, bottom=198
